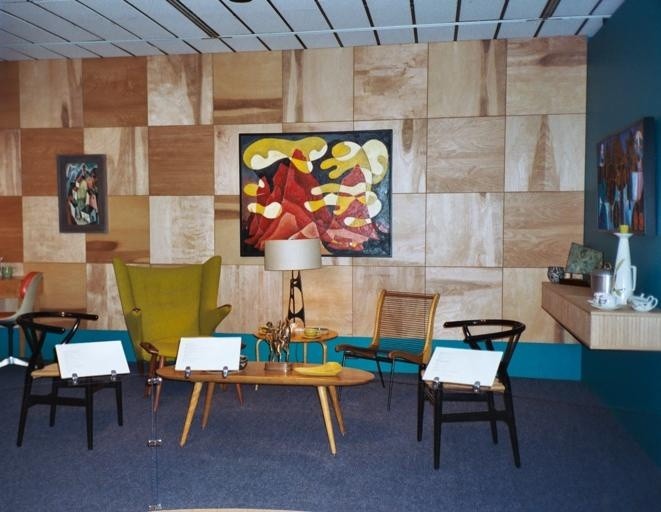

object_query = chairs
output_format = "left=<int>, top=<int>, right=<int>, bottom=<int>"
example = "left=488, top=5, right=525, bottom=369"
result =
left=334, top=288, right=441, bottom=412
left=416, top=319, right=526, bottom=470
left=16, top=311, right=123, bottom=450
left=0, top=272, right=44, bottom=367
left=112, top=256, right=232, bottom=397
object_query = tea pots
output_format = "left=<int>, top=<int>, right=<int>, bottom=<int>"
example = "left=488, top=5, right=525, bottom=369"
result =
left=613, top=232, right=636, bottom=304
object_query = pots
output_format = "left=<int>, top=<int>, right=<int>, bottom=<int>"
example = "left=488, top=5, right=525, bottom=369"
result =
left=590, top=271, right=613, bottom=297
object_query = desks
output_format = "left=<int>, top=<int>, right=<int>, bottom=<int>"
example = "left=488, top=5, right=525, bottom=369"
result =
left=252, top=326, right=339, bottom=391
left=154, top=362, right=374, bottom=455
left=542, top=283, right=661, bottom=352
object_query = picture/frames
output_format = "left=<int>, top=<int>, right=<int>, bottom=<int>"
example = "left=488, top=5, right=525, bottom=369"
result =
left=56, top=154, right=109, bottom=234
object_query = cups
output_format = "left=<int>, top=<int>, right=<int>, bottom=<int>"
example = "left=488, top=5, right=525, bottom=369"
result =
left=632, top=294, right=658, bottom=312
left=2, top=266, right=12, bottom=279
left=595, top=293, right=616, bottom=306
left=304, top=328, right=318, bottom=337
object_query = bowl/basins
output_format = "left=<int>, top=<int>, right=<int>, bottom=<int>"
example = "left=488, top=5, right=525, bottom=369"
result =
left=240, top=356, right=249, bottom=371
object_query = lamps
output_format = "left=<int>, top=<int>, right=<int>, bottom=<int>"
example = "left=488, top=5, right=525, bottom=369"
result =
left=264, top=239, right=323, bottom=328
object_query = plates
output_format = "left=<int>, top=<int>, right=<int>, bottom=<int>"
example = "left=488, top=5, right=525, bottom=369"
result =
left=293, top=361, right=343, bottom=376
left=585, top=300, right=622, bottom=310
left=302, top=335, right=320, bottom=339
